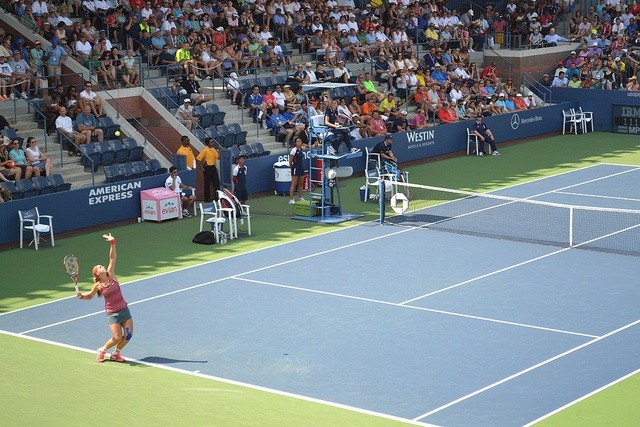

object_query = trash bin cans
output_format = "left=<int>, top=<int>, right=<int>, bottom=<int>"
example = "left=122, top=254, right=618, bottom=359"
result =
left=359, top=188, right=370, bottom=202
left=210, top=222, right=223, bottom=232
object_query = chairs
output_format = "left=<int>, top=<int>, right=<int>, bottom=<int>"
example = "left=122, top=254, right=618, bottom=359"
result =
left=573, top=106, right=594, bottom=133
left=165, top=182, right=198, bottom=219
left=282, top=75, right=292, bottom=89
left=249, top=78, right=262, bottom=95
left=170, top=109, right=177, bottom=115
left=103, top=165, right=125, bottom=182
left=364, top=167, right=394, bottom=203
left=217, top=124, right=234, bottom=147
left=345, top=86, right=360, bottom=101
left=206, top=103, right=226, bottom=125
left=191, top=128, right=212, bottom=150
left=365, top=146, right=386, bottom=171
left=466, top=127, right=492, bottom=156
left=88, top=117, right=105, bottom=143
left=119, top=163, right=140, bottom=178
left=205, top=126, right=224, bottom=147
left=1, top=181, right=24, bottom=199
left=34, top=101, right=52, bottom=129
left=225, top=81, right=233, bottom=99
left=229, top=145, right=246, bottom=162
left=272, top=76, right=282, bottom=91
left=177, top=88, right=190, bottom=107
left=228, top=122, right=248, bottom=145
left=242, top=79, right=253, bottom=97
left=16, top=178, right=40, bottom=196
left=262, top=76, right=274, bottom=93
left=308, top=114, right=344, bottom=155
left=149, top=89, right=170, bottom=107
left=48, top=173, right=72, bottom=191
left=1, top=128, right=24, bottom=148
left=108, top=139, right=129, bottom=163
left=196, top=105, right=213, bottom=127
left=60, top=121, right=80, bottom=151
left=239, top=144, right=258, bottom=157
left=161, top=88, right=179, bottom=107
left=191, top=107, right=201, bottom=128
left=218, top=199, right=252, bottom=238
left=73, top=120, right=92, bottom=144
left=147, top=158, right=169, bottom=174
left=250, top=142, right=271, bottom=155
left=273, top=127, right=286, bottom=142
left=561, top=109, right=585, bottom=134
left=94, top=140, right=115, bottom=167
left=132, top=160, right=154, bottom=177
left=32, top=175, right=55, bottom=193
left=336, top=87, right=350, bottom=103
left=198, top=200, right=234, bottom=244
left=99, top=114, right=121, bottom=140
left=122, top=137, right=144, bottom=161
left=78, top=143, right=101, bottom=172
left=18, top=206, right=56, bottom=251
left=248, top=104, right=259, bottom=123
left=383, top=165, right=410, bottom=199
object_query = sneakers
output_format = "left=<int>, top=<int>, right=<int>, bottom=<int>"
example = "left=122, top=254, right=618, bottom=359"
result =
left=96, top=346, right=106, bottom=364
left=109, top=352, right=126, bottom=362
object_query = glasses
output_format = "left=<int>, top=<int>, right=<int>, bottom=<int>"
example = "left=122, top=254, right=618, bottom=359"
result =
left=173, top=171, right=177, bottom=173
left=210, top=141, right=213, bottom=143
left=86, top=86, right=91, bottom=87
left=341, top=101, right=346, bottom=103
left=31, top=141, right=37, bottom=143
left=13, top=142, right=19, bottom=145
left=353, top=98, right=358, bottom=100
left=186, top=139, right=190, bottom=141
left=186, top=102, right=191, bottom=104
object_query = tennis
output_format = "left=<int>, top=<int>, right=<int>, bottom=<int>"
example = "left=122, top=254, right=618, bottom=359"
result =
left=115, top=131, right=120, bottom=137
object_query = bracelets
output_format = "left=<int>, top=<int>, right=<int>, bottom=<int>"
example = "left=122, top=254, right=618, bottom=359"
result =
left=110, top=239, right=116, bottom=245
left=79, top=295, right=83, bottom=300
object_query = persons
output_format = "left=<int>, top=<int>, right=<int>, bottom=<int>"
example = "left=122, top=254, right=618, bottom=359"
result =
left=165, top=1, right=323, bottom=132
left=469, top=113, right=501, bottom=157
left=175, top=135, right=196, bottom=171
left=370, top=134, right=400, bottom=181
left=0, top=1, right=164, bottom=203
left=285, top=132, right=322, bottom=147
left=288, top=137, right=305, bottom=205
left=76, top=232, right=133, bottom=363
left=323, top=0, right=469, bottom=98
left=323, top=98, right=361, bottom=156
left=361, top=98, right=469, bottom=139
left=195, top=138, right=222, bottom=203
left=469, top=0, right=640, bottom=113
left=165, top=167, right=198, bottom=217
left=232, top=153, right=248, bottom=213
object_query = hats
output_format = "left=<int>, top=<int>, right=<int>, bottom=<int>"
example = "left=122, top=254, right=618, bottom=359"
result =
left=216, top=26, right=224, bottom=31
left=527, top=92, right=533, bottom=97
left=285, top=104, right=296, bottom=110
left=477, top=113, right=482, bottom=117
left=468, top=9, right=474, bottom=16
left=13, top=50, right=20, bottom=55
left=184, top=98, right=191, bottom=102
left=385, top=134, right=394, bottom=140
left=266, top=86, right=273, bottom=90
left=230, top=72, right=238, bottom=79
left=232, top=12, right=238, bottom=17
left=499, top=93, right=504, bottom=98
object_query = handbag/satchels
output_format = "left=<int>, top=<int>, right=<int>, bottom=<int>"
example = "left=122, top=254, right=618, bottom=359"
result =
left=192, top=231, right=214, bottom=244
